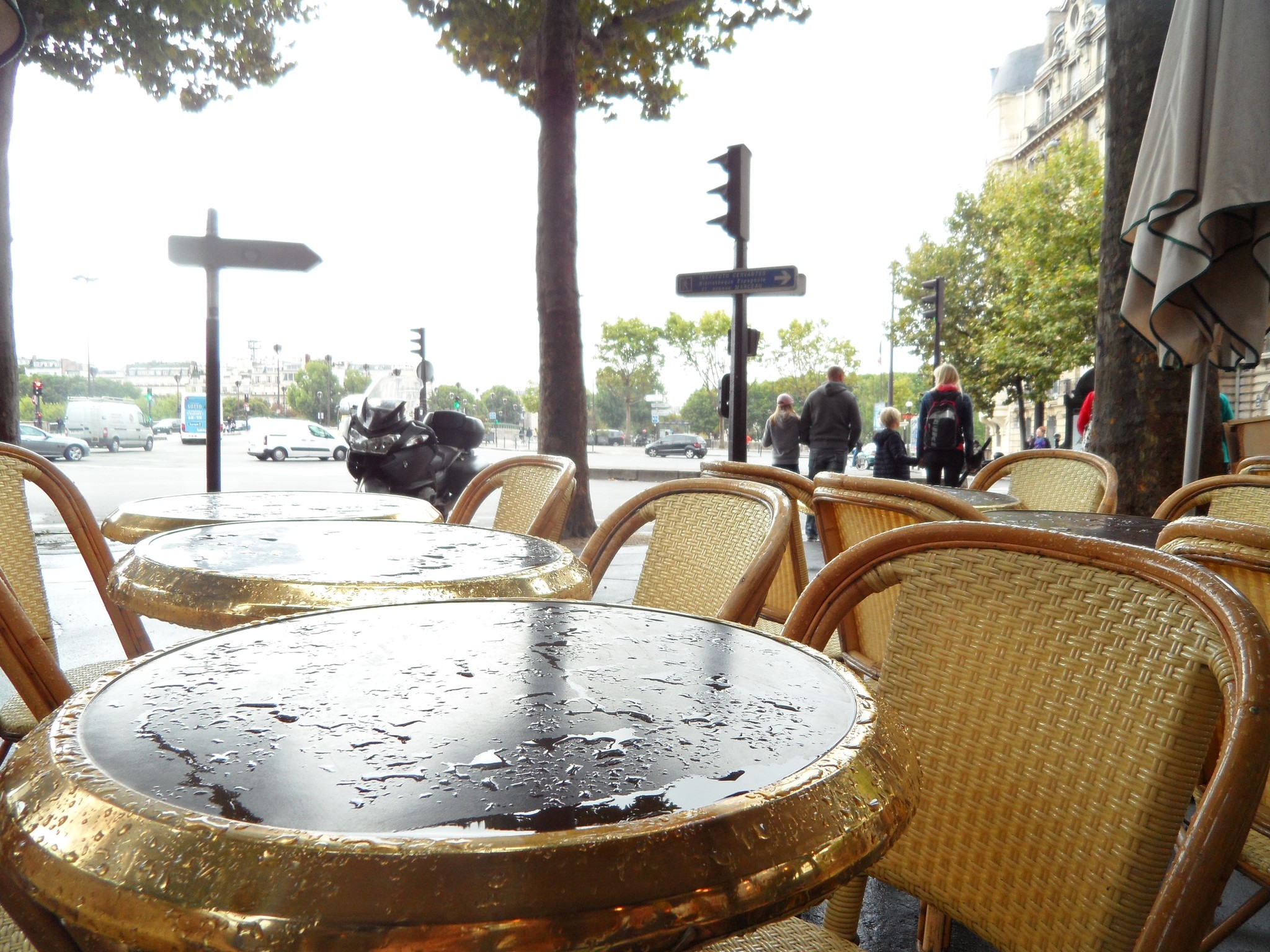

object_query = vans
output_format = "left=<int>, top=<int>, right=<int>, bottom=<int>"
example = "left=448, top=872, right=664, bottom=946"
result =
left=63, top=396, right=155, bottom=453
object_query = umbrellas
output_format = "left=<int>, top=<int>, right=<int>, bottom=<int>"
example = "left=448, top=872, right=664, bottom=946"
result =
left=1120, top=0, right=1270, bottom=517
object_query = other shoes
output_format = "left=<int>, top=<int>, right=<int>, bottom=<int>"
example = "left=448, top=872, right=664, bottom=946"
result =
left=808, top=536, right=820, bottom=542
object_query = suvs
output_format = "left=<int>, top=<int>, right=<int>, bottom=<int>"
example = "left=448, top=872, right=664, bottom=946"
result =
left=644, top=434, right=708, bottom=459
left=586, top=429, right=625, bottom=446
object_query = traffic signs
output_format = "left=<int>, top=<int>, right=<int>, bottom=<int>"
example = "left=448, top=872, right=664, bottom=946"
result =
left=675, top=265, right=799, bottom=297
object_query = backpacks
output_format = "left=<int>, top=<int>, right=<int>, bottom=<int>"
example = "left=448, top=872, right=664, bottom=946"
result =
left=922, top=389, right=964, bottom=452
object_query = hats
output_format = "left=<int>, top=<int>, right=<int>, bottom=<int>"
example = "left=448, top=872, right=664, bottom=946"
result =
left=973, top=440, right=979, bottom=444
left=777, top=393, right=794, bottom=406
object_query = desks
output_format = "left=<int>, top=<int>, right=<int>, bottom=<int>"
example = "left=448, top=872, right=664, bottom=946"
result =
left=924, top=484, right=1029, bottom=514
left=0, top=599, right=923, bottom=952
left=103, top=491, right=442, bottom=545
left=105, top=521, right=593, bottom=633
left=982, top=510, right=1175, bottom=551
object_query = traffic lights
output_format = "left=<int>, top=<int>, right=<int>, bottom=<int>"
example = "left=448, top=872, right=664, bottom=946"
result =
left=33, top=382, right=36, bottom=395
left=455, top=396, right=460, bottom=409
left=146, top=388, right=152, bottom=401
left=36, top=382, right=43, bottom=395
left=922, top=276, right=944, bottom=321
left=409, top=328, right=424, bottom=357
left=705, top=143, right=743, bottom=241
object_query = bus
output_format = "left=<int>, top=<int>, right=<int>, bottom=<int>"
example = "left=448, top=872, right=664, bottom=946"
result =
left=180, top=392, right=224, bottom=445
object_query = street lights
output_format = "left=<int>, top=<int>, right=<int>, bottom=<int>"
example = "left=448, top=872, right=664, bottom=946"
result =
left=280, top=385, right=287, bottom=416
left=317, top=391, right=323, bottom=424
left=234, top=380, right=241, bottom=419
left=173, top=373, right=182, bottom=415
left=325, top=354, right=334, bottom=429
left=363, top=364, right=369, bottom=389
left=273, top=343, right=283, bottom=417
left=449, top=381, right=525, bottom=447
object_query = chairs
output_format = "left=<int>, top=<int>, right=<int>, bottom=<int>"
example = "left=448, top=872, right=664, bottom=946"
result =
left=0, top=414, right=1270, bottom=952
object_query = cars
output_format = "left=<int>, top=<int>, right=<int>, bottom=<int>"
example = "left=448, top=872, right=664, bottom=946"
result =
left=224, top=421, right=250, bottom=431
left=20, top=423, right=90, bottom=463
left=152, top=418, right=180, bottom=435
left=247, top=417, right=350, bottom=462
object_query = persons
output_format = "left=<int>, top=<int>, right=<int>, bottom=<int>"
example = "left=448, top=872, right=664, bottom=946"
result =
left=762, top=393, right=801, bottom=474
left=1026, top=426, right=1053, bottom=449
left=1077, top=391, right=1094, bottom=437
left=916, top=365, right=986, bottom=487
left=849, top=439, right=863, bottom=468
left=799, top=366, right=862, bottom=542
left=873, top=407, right=922, bottom=482
left=227, top=416, right=236, bottom=433
left=1220, top=392, right=1235, bottom=474
left=519, top=427, right=538, bottom=444
left=55, top=417, right=63, bottom=434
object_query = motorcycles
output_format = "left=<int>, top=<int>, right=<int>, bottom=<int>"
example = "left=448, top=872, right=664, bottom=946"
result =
left=337, top=373, right=494, bottom=522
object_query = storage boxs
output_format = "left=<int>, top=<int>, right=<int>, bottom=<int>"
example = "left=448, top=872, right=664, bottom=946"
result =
left=422, top=410, right=485, bottom=450
left=447, top=453, right=489, bottom=496
